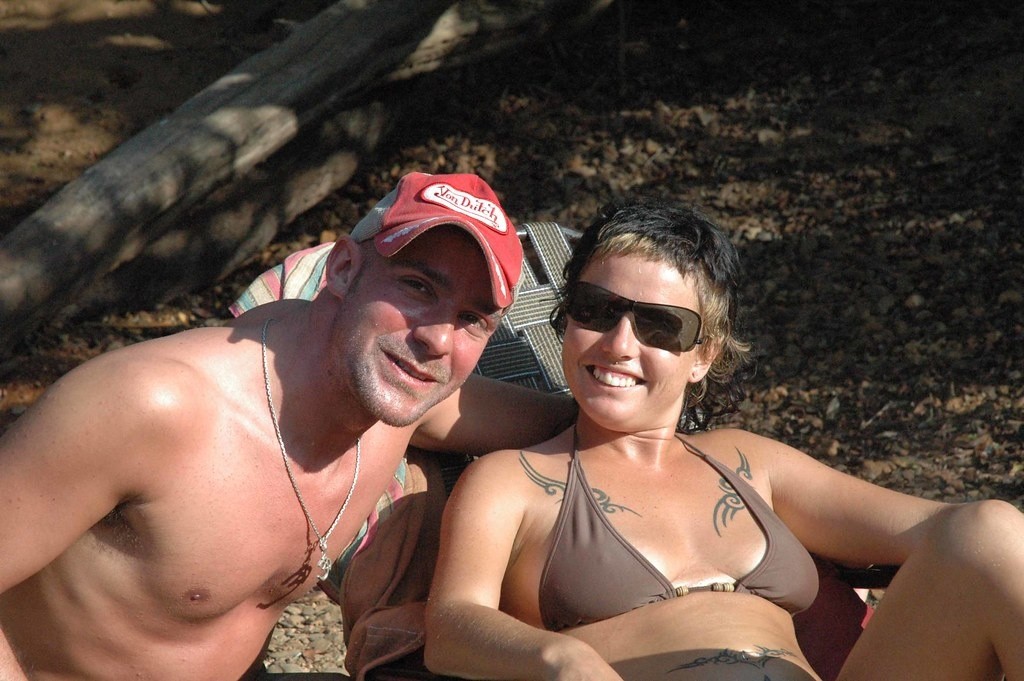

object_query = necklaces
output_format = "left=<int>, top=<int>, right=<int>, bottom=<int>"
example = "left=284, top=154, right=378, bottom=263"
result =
left=262, top=317, right=361, bottom=580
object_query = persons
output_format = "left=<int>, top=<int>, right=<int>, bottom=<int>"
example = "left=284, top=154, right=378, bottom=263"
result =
left=421, top=192, right=1024, bottom=680
left=0, top=173, right=580, bottom=681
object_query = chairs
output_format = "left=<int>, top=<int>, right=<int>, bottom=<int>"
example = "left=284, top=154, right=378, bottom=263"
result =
left=226, top=222, right=901, bottom=681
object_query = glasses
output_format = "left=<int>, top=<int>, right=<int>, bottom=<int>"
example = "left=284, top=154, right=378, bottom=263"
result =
left=564, top=281, right=707, bottom=352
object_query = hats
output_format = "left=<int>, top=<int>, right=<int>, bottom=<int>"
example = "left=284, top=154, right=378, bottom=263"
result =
left=350, top=171, right=523, bottom=308
